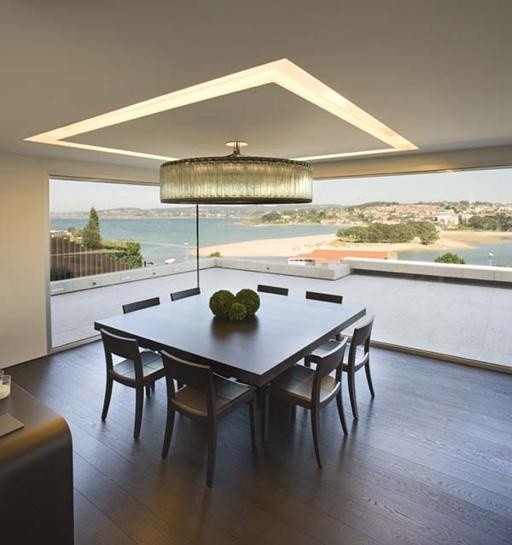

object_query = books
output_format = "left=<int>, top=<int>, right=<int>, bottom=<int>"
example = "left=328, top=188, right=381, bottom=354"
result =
left=0, top=411, right=26, bottom=439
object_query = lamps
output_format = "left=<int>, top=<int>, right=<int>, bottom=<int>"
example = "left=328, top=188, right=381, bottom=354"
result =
left=158, top=140, right=316, bottom=206
